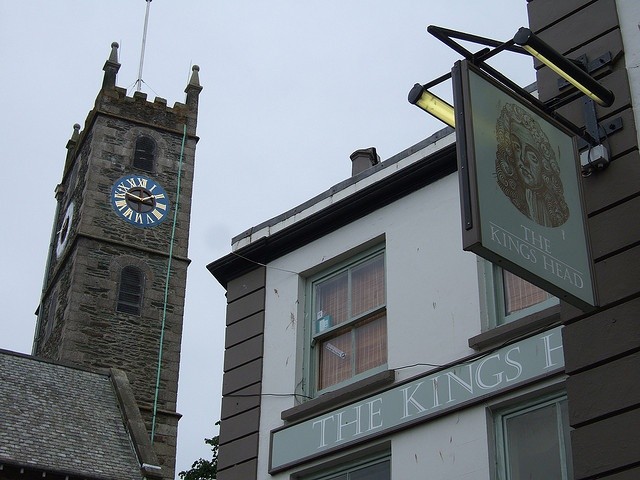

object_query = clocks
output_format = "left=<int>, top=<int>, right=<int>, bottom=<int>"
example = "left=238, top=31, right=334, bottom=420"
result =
left=115, top=177, right=167, bottom=224
left=56, top=201, right=76, bottom=260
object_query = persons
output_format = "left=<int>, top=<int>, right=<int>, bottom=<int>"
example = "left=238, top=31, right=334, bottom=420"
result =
left=495, top=102, right=570, bottom=228
